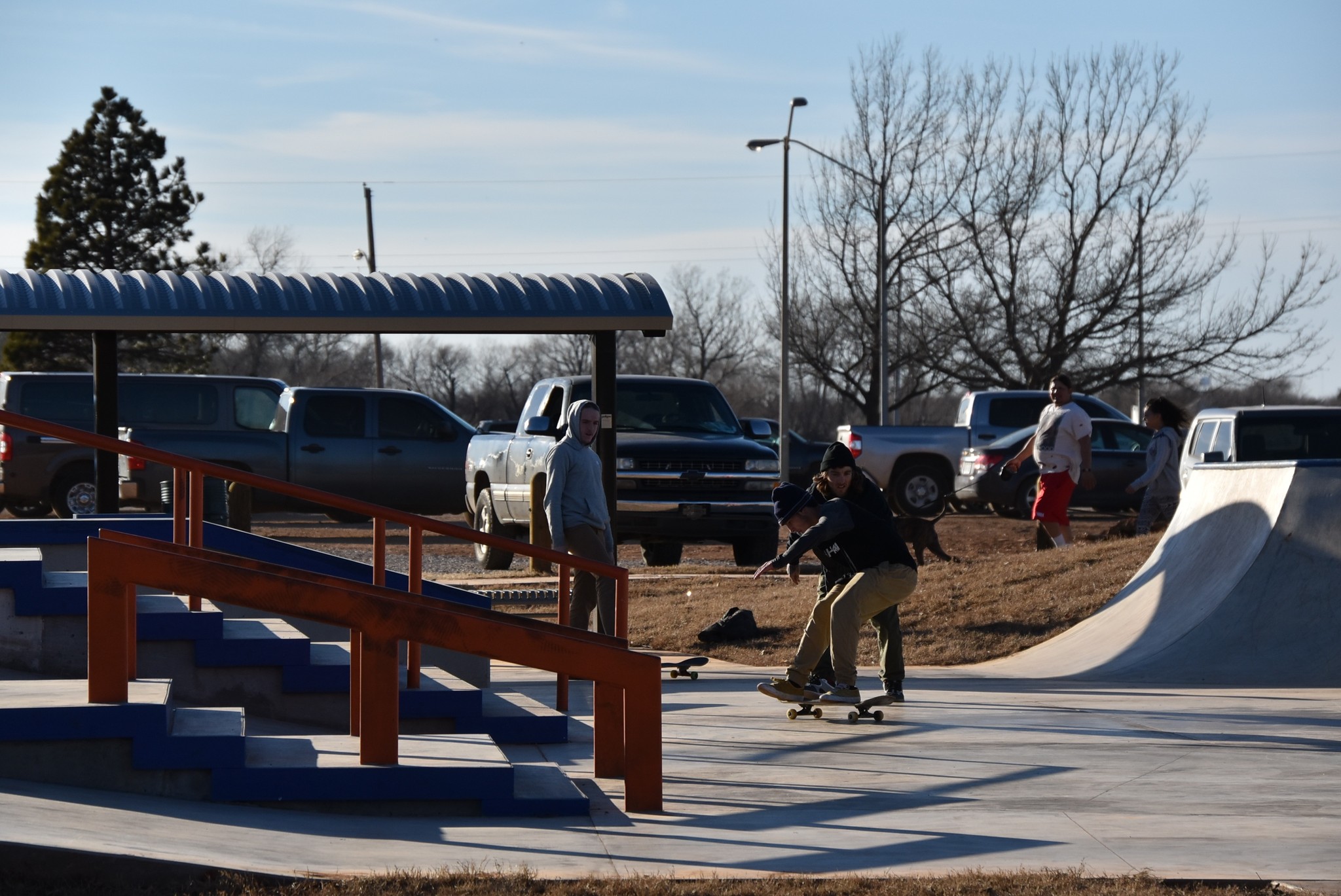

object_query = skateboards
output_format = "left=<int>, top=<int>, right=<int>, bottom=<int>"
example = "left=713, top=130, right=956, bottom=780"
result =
left=661, top=657, right=708, bottom=680
left=773, top=694, right=897, bottom=723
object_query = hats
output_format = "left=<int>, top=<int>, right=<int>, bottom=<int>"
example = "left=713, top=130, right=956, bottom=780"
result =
left=772, top=481, right=812, bottom=526
left=820, top=441, right=856, bottom=475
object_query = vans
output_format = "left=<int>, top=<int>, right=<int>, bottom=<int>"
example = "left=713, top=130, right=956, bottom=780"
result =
left=1179, top=405, right=1340, bottom=481
left=0, top=371, right=327, bottom=519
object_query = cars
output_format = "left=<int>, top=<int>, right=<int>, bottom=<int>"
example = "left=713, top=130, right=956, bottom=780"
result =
left=737, top=417, right=840, bottom=483
left=955, top=419, right=1153, bottom=518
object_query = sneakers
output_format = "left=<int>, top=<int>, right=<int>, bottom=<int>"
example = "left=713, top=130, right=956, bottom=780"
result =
left=883, top=680, right=904, bottom=702
left=819, top=685, right=861, bottom=704
left=757, top=676, right=805, bottom=702
left=805, top=674, right=837, bottom=700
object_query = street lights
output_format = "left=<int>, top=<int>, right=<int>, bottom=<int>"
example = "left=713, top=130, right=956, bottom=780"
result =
left=355, top=182, right=384, bottom=389
left=775, top=97, right=812, bottom=479
left=746, top=138, right=896, bottom=428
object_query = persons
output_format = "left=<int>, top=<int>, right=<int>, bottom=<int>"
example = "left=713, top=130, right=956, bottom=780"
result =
left=1126, top=396, right=1192, bottom=535
left=543, top=399, right=615, bottom=681
left=785, top=441, right=906, bottom=703
left=1004, top=374, right=1097, bottom=548
left=752, top=481, right=918, bottom=700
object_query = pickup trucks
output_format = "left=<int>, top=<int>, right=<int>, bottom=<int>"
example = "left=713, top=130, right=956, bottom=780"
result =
left=463, top=376, right=789, bottom=567
left=831, top=389, right=1139, bottom=520
left=115, top=383, right=500, bottom=524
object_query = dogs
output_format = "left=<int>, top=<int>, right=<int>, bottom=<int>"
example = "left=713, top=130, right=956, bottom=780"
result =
left=889, top=492, right=964, bottom=567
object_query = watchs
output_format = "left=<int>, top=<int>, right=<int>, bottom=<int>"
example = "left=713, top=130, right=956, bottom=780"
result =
left=1082, top=468, right=1091, bottom=472
